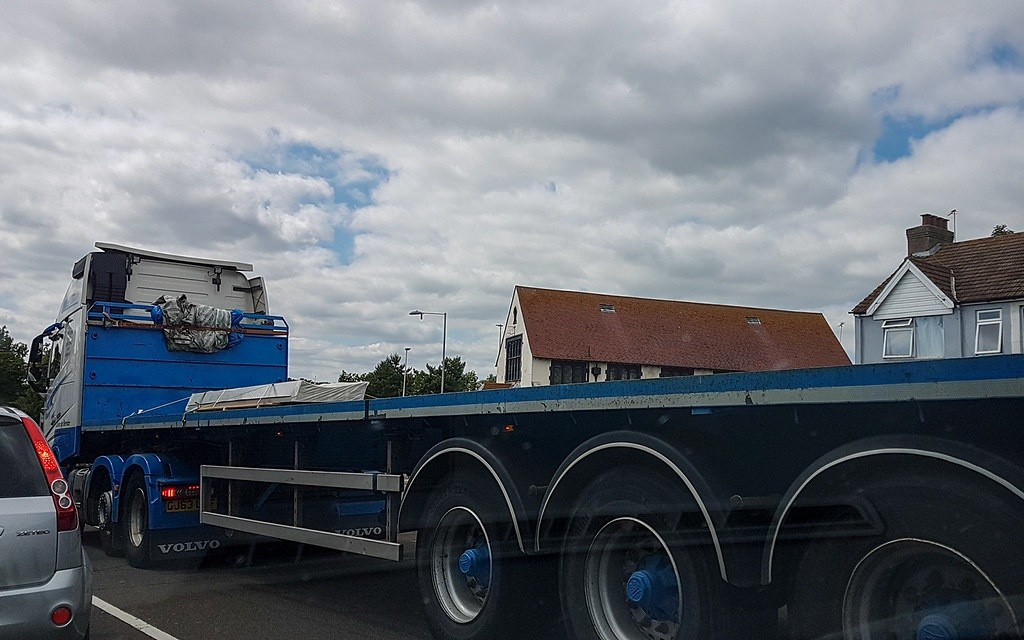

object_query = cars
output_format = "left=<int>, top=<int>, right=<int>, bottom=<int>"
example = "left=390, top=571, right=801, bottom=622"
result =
left=0, top=405, right=95, bottom=640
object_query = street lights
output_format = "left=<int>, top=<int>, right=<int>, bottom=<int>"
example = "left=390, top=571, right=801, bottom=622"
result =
left=409, top=309, right=447, bottom=394
left=402, top=347, right=412, bottom=397
left=837, top=322, right=845, bottom=342
left=496, top=323, right=503, bottom=344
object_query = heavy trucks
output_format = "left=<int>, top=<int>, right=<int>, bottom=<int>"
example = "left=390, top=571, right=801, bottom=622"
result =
left=26, top=239, right=1023, bottom=640
